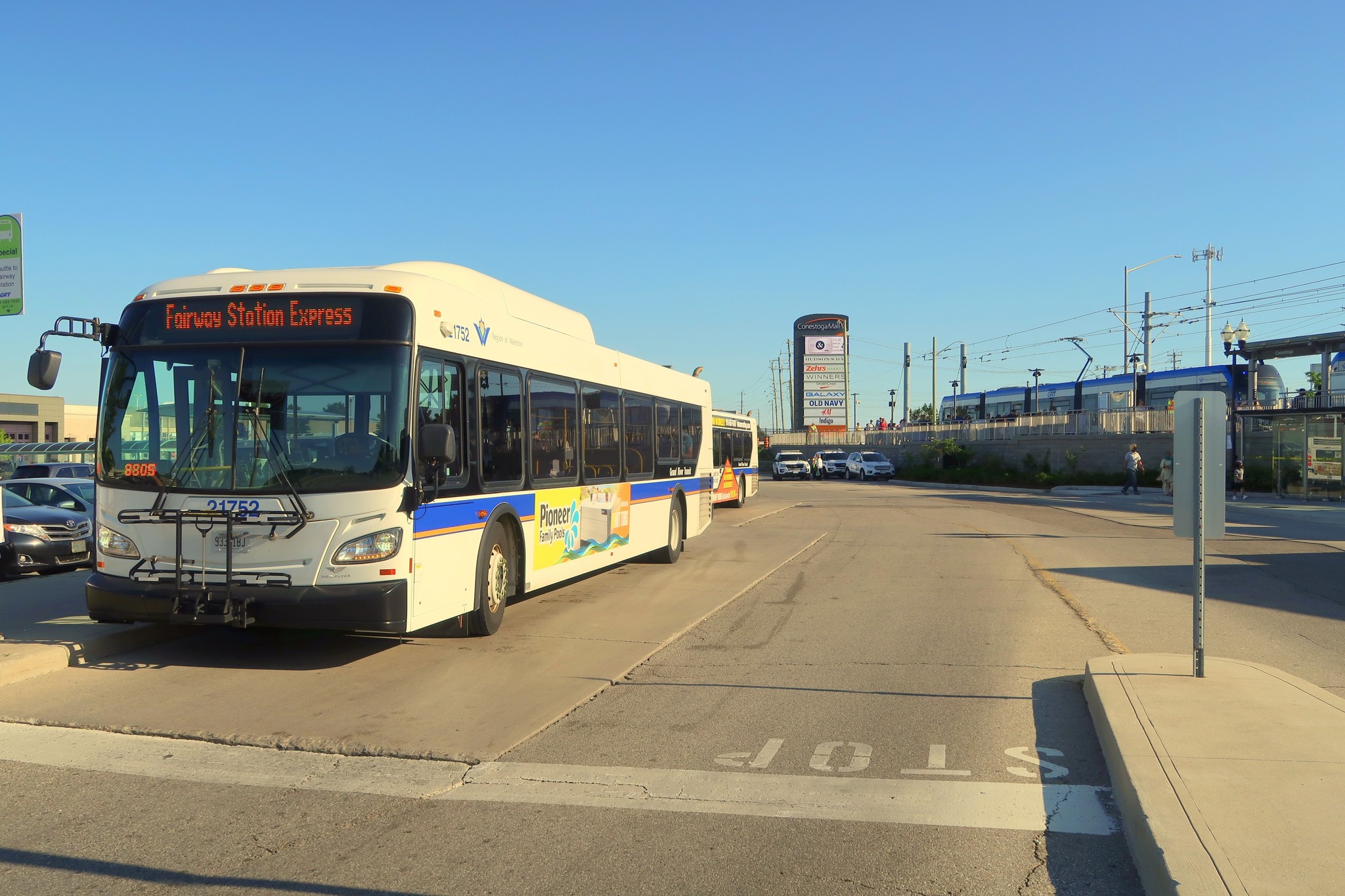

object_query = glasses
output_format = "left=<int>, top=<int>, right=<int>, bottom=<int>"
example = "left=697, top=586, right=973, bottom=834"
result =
left=1133, top=448, right=1137, bottom=449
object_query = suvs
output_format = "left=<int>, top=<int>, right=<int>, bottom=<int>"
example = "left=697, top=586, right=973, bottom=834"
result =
left=772, top=450, right=811, bottom=481
left=844, top=450, right=895, bottom=482
left=812, top=450, right=850, bottom=480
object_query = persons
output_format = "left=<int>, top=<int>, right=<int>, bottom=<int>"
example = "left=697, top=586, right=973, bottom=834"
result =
left=813, top=453, right=819, bottom=480
left=1160, top=451, right=1173, bottom=496
left=986, top=409, right=1020, bottom=440
left=1121, top=444, right=1145, bottom=495
left=1023, top=409, right=1048, bottom=435
left=855, top=423, right=863, bottom=445
left=867, top=419, right=874, bottom=444
left=1235, top=398, right=1263, bottom=432
left=678, top=428, right=692, bottom=459
left=897, top=414, right=932, bottom=444
left=816, top=455, right=823, bottom=481
left=809, top=422, right=818, bottom=445
left=1232, top=461, right=1248, bottom=500
left=1167, top=400, right=1174, bottom=433
left=1136, top=400, right=1151, bottom=434
left=945, top=410, right=972, bottom=441
left=864, top=424, right=869, bottom=444
left=1050, top=407, right=1059, bottom=434
left=1091, top=412, right=1098, bottom=434
left=1291, top=388, right=1308, bottom=408
left=875, top=417, right=897, bottom=445
left=1314, top=385, right=1331, bottom=408
left=1064, top=410, right=1075, bottom=435
left=1078, top=408, right=1088, bottom=434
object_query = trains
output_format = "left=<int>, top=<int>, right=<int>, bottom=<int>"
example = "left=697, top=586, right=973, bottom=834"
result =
left=938, top=336, right=1288, bottom=431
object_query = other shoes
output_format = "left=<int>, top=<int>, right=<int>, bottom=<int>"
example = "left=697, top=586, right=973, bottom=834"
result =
left=1243, top=495, right=1248, bottom=500
left=1233, top=496, right=1237, bottom=500
left=1134, top=492, right=1140, bottom=495
left=1121, top=490, right=1129, bottom=495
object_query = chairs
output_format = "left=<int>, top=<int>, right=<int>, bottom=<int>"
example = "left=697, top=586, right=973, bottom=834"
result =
left=492, top=432, right=698, bottom=478
left=57, top=500, right=75, bottom=508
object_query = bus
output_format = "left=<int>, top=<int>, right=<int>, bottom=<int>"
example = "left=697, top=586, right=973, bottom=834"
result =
left=23, top=259, right=716, bottom=639
left=712, top=408, right=760, bottom=509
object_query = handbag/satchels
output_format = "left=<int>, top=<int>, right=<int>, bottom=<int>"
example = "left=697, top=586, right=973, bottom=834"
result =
left=810, top=464, right=816, bottom=473
left=816, top=469, right=820, bottom=476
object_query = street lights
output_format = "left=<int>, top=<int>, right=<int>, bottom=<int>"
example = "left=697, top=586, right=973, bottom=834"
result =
left=1122, top=254, right=1184, bottom=375
left=1220, top=317, right=1250, bottom=489
left=931, top=339, right=965, bottom=425
left=1027, top=368, right=1046, bottom=414
left=850, top=393, right=860, bottom=430
left=948, top=380, right=962, bottom=420
left=887, top=389, right=898, bottom=424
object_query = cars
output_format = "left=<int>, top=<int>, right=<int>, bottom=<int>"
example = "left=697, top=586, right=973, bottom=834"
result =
left=0, top=477, right=96, bottom=581
left=6, top=461, right=97, bottom=503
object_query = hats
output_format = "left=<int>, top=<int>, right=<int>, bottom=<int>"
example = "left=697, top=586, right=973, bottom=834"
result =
left=1129, top=444, right=1137, bottom=450
